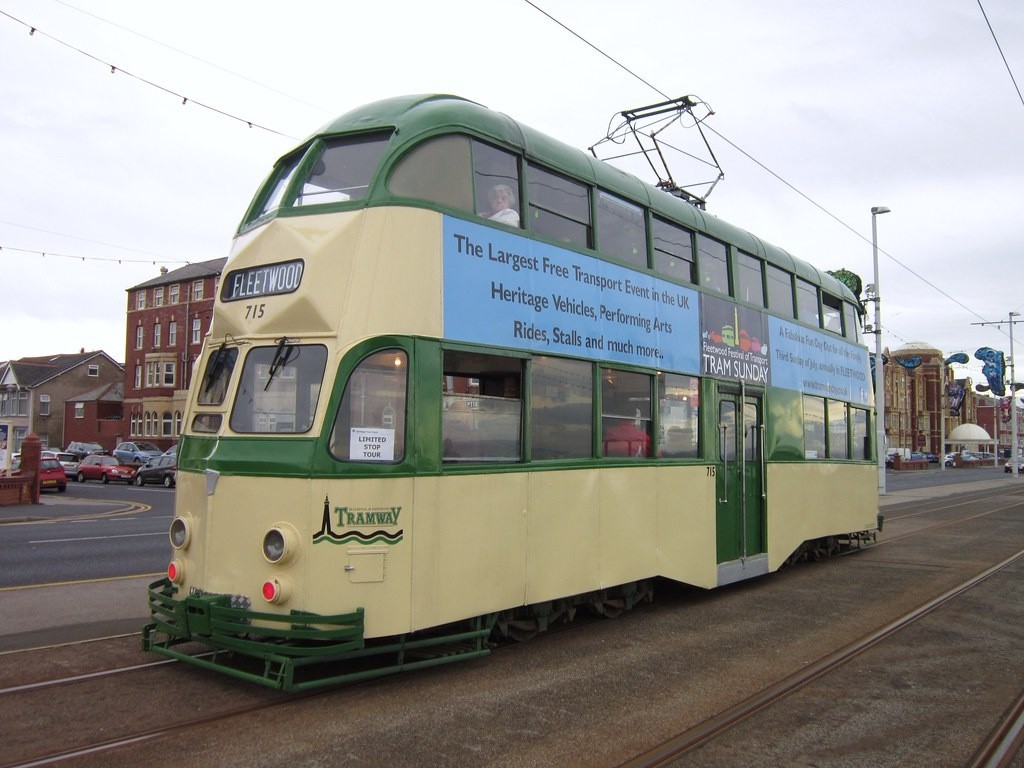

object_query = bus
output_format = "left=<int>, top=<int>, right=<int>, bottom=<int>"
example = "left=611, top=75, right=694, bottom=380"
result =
left=132, top=88, right=890, bottom=703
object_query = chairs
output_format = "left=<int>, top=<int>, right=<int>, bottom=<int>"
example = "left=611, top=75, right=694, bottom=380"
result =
left=448, top=401, right=866, bottom=462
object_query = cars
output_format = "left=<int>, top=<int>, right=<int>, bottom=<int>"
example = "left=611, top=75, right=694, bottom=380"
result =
left=74, top=455, right=135, bottom=487
left=64, top=441, right=110, bottom=461
left=885, top=449, right=1009, bottom=472
left=161, top=445, right=177, bottom=458
left=1004, top=456, right=1024, bottom=473
left=111, top=441, right=163, bottom=466
left=11, top=453, right=21, bottom=465
left=12, top=457, right=67, bottom=493
left=133, top=455, right=176, bottom=488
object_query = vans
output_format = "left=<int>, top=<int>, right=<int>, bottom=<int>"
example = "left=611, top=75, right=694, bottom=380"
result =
left=40, top=450, right=80, bottom=480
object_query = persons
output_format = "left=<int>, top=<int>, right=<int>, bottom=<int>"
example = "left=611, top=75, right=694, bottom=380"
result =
left=440, top=402, right=490, bottom=464
left=601, top=379, right=620, bottom=422
left=476, top=183, right=520, bottom=228
left=602, top=404, right=664, bottom=458
